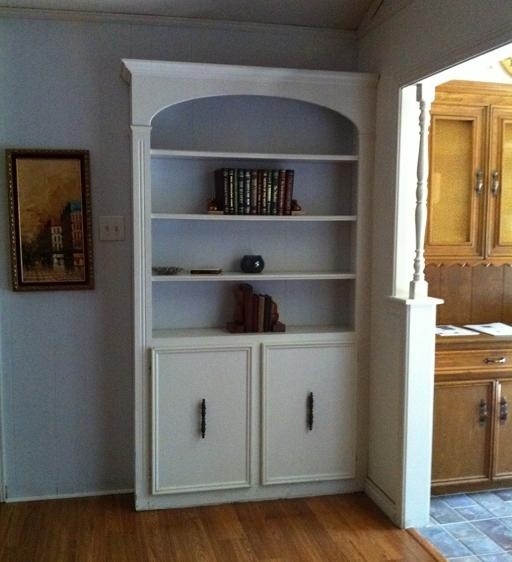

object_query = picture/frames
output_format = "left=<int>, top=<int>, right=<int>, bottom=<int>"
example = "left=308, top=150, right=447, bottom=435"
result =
left=5, top=148, right=96, bottom=292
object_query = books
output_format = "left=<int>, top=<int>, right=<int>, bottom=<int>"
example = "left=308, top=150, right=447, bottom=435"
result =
left=216, top=166, right=295, bottom=215
left=435, top=324, right=480, bottom=337
left=235, top=283, right=278, bottom=331
left=462, top=321, right=511, bottom=337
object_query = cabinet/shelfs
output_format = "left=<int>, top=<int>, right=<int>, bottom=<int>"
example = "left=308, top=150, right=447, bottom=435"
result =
left=431, top=337, right=512, bottom=495
left=425, top=80, right=512, bottom=267
left=120, top=56, right=380, bottom=510
